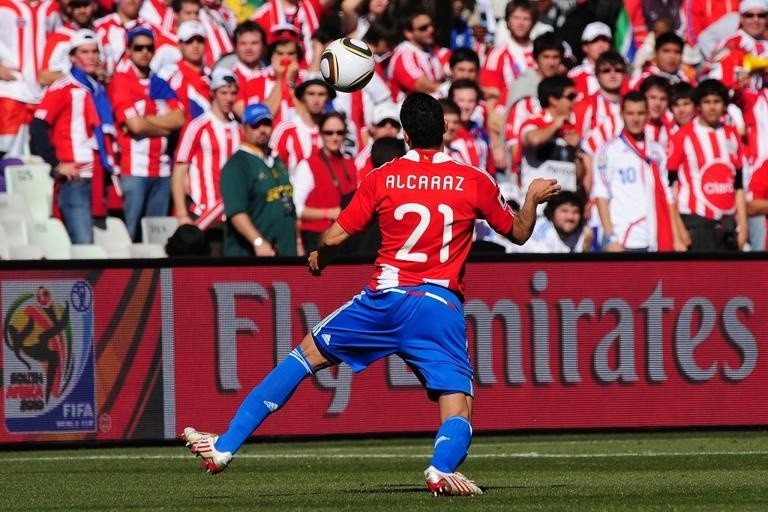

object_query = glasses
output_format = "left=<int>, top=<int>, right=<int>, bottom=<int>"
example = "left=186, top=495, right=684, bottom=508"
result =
left=320, top=130, right=345, bottom=136
left=744, top=12, right=766, bottom=18
left=414, top=23, right=432, bottom=31
left=563, top=92, right=578, bottom=101
left=598, top=66, right=625, bottom=73
left=134, top=44, right=154, bottom=52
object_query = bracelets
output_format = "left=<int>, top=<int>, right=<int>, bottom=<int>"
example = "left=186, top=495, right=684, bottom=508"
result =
left=323, top=208, right=329, bottom=220
left=286, top=80, right=297, bottom=89
left=604, top=233, right=618, bottom=246
left=53, top=161, right=65, bottom=171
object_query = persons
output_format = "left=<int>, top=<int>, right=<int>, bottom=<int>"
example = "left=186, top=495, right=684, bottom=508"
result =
left=105, top=20, right=186, bottom=242
left=667, top=82, right=695, bottom=136
left=387, top=13, right=446, bottom=102
left=566, top=22, right=612, bottom=117
left=342, top=14, right=404, bottom=131
left=267, top=70, right=337, bottom=170
left=591, top=90, right=686, bottom=253
left=29, top=27, right=124, bottom=245
left=290, top=111, right=357, bottom=257
left=226, top=19, right=265, bottom=107
left=94, top=0, right=160, bottom=68
left=436, top=98, right=466, bottom=165
left=513, top=73, right=585, bottom=193
left=219, top=103, right=308, bottom=258
left=339, top=0, right=392, bottom=39
left=559, top=51, right=627, bottom=156
left=37, top=0, right=100, bottom=87
left=155, top=20, right=216, bottom=123
left=745, top=148, right=768, bottom=253
left=633, top=18, right=694, bottom=75
left=366, top=137, right=406, bottom=255
left=637, top=75, right=670, bottom=176
left=434, top=1, right=478, bottom=49
left=539, top=0, right=690, bottom=30
left=252, top=0, right=334, bottom=42
left=243, top=23, right=320, bottom=109
left=480, top=1, right=539, bottom=105
left=450, top=47, right=480, bottom=82
left=700, top=0, right=768, bottom=83
left=180, top=90, right=562, bottom=498
left=443, top=79, right=498, bottom=183
left=630, top=32, right=699, bottom=91
left=170, top=66, right=249, bottom=256
left=678, top=0, right=739, bottom=39
left=0, top=0, right=64, bottom=155
left=666, top=79, right=750, bottom=251
left=503, top=31, right=563, bottom=109
left=511, top=190, right=596, bottom=254
left=357, top=116, right=401, bottom=182
left=166, top=0, right=214, bottom=69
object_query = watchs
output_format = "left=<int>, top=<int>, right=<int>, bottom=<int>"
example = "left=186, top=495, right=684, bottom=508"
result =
left=250, top=234, right=266, bottom=248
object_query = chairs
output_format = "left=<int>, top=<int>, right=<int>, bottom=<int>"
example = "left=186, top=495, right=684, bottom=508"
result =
left=0, top=153, right=185, bottom=259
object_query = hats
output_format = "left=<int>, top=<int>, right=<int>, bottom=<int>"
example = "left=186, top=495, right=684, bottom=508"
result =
left=582, top=21, right=613, bottom=41
left=739, top=1, right=768, bottom=14
left=266, top=22, right=303, bottom=45
left=242, top=104, right=274, bottom=125
left=372, top=101, right=402, bottom=128
left=69, top=28, right=98, bottom=51
left=209, top=66, right=238, bottom=90
left=177, top=20, right=206, bottom=42
left=294, top=72, right=337, bottom=99
left=127, top=26, right=153, bottom=41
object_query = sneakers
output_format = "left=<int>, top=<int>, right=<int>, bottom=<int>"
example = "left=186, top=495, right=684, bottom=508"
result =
left=423, top=465, right=482, bottom=497
left=181, top=427, right=234, bottom=473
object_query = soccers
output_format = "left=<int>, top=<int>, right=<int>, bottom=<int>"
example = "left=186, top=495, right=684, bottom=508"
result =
left=320, top=38, right=375, bottom=94
